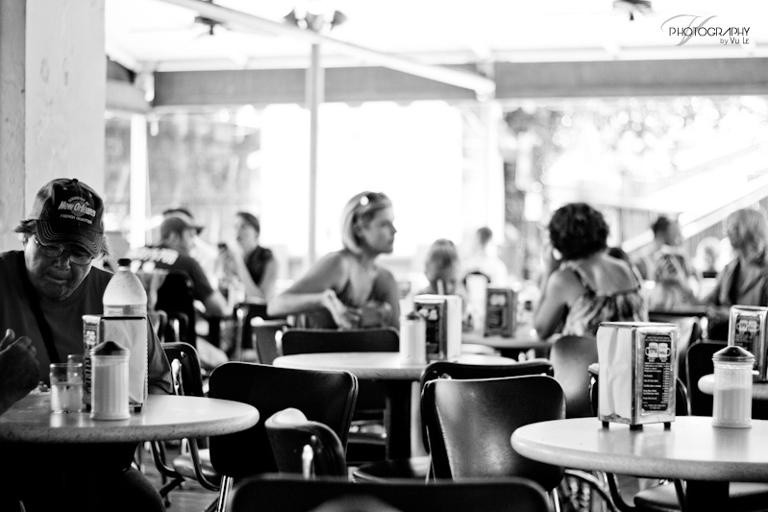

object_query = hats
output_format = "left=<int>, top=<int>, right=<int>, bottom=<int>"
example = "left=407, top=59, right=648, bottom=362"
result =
left=15, top=178, right=104, bottom=256
left=158, top=218, right=202, bottom=236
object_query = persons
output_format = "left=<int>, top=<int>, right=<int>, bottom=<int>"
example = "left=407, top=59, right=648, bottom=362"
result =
left=266, top=192, right=400, bottom=330
left=215, top=213, right=275, bottom=300
left=531, top=205, right=650, bottom=341
left=0, top=179, right=165, bottom=512
left=468, top=227, right=506, bottom=279
left=125, top=214, right=245, bottom=370
left=705, top=208, right=767, bottom=309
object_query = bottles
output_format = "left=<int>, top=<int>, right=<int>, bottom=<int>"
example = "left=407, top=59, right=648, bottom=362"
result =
left=710, top=345, right=755, bottom=429
left=103, top=258, right=148, bottom=407
left=397, top=310, right=426, bottom=365
left=90, top=339, right=132, bottom=422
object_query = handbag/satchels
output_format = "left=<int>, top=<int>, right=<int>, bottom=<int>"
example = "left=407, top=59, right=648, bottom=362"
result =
left=59, top=442, right=140, bottom=485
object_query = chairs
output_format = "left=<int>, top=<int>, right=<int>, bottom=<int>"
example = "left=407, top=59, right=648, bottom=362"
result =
left=225, top=478, right=553, bottom=511
left=423, top=374, right=565, bottom=492
left=230, top=302, right=274, bottom=357
left=151, top=343, right=222, bottom=511
left=282, top=330, right=400, bottom=354
left=686, top=341, right=729, bottom=416
left=587, top=374, right=768, bottom=512
left=547, top=333, right=599, bottom=418
left=252, top=317, right=291, bottom=364
left=209, top=362, right=359, bottom=512
left=266, top=407, right=350, bottom=478
left=353, top=361, right=556, bottom=481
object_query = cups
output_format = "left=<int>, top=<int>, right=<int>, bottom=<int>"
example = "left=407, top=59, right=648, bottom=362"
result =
left=65, top=354, right=83, bottom=384
left=727, top=305, right=767, bottom=381
left=47, top=360, right=87, bottom=417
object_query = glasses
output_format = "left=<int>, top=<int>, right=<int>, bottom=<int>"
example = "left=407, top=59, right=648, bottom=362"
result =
left=359, top=193, right=389, bottom=204
left=29, top=231, right=95, bottom=264
